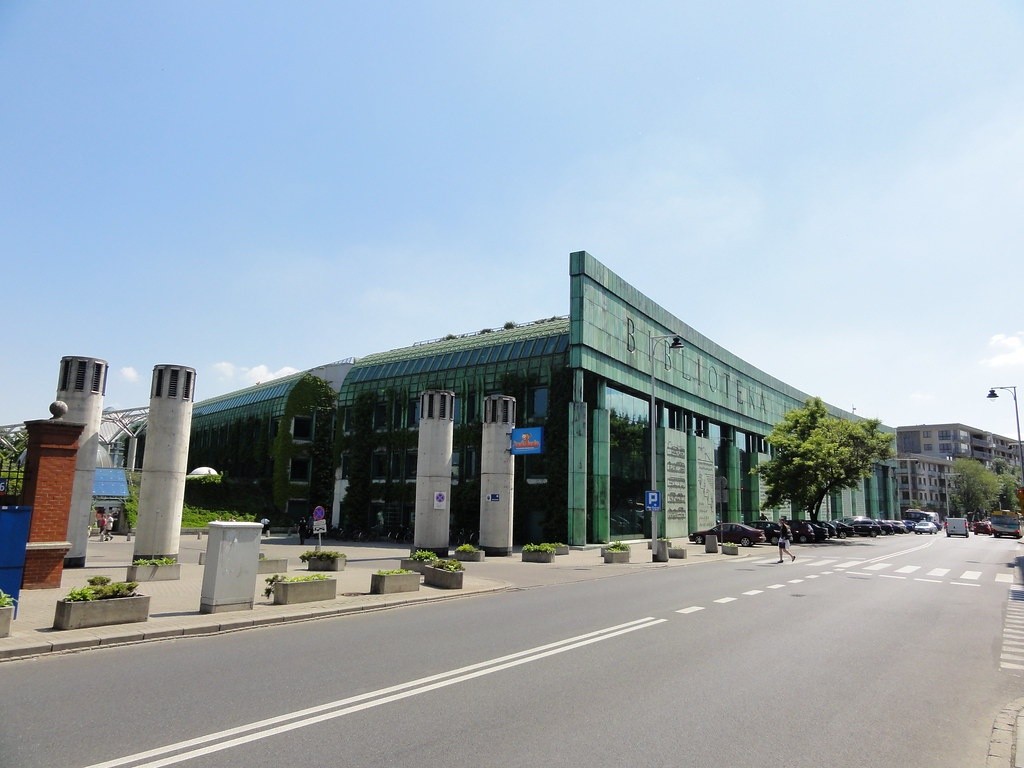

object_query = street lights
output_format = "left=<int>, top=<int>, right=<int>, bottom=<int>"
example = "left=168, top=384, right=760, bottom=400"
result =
left=986, top=385, right=1024, bottom=487
left=648, top=329, right=685, bottom=564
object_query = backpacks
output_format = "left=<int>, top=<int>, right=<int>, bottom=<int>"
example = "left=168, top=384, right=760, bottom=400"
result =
left=785, top=525, right=792, bottom=540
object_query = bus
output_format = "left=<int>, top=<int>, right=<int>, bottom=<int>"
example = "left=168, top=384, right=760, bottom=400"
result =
left=904, top=508, right=939, bottom=525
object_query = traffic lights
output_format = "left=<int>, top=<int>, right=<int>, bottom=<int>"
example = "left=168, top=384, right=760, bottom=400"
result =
left=1015, top=488, right=1024, bottom=500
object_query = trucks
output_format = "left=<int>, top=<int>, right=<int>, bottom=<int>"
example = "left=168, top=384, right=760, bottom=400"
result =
left=991, top=501, right=1024, bottom=540
left=944, top=518, right=970, bottom=538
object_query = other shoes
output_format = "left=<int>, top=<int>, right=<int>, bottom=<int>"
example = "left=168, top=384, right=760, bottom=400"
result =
left=777, top=560, right=784, bottom=563
left=105, top=536, right=113, bottom=540
left=791, top=556, right=795, bottom=562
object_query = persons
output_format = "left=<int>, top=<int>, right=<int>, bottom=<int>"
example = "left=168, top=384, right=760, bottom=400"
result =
left=777, top=518, right=795, bottom=563
left=759, top=513, right=768, bottom=521
left=261, top=517, right=270, bottom=534
left=297, top=516, right=308, bottom=545
left=95, top=513, right=114, bottom=542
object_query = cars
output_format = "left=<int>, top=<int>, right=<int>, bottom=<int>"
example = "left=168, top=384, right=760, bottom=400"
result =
left=973, top=521, right=992, bottom=535
left=688, top=522, right=767, bottom=548
left=610, top=512, right=643, bottom=533
left=785, top=515, right=918, bottom=544
left=932, top=521, right=943, bottom=531
left=735, top=519, right=793, bottom=546
left=914, top=521, right=939, bottom=535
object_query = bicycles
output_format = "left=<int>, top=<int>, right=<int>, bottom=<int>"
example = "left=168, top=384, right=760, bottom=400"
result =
left=296, top=514, right=414, bottom=545
left=449, top=526, right=480, bottom=547
